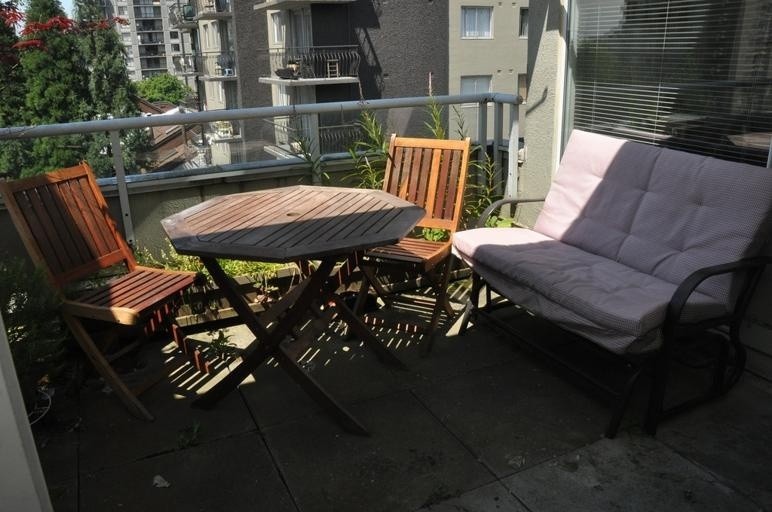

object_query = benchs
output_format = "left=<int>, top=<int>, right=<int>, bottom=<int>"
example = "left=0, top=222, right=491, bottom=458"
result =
left=451, top=129, right=772, bottom=440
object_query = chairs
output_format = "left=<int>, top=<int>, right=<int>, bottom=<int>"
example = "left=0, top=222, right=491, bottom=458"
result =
left=345, top=134, right=472, bottom=357
left=0, top=160, right=215, bottom=423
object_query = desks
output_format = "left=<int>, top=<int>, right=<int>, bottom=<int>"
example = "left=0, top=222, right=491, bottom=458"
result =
left=161, top=184, right=426, bottom=436
